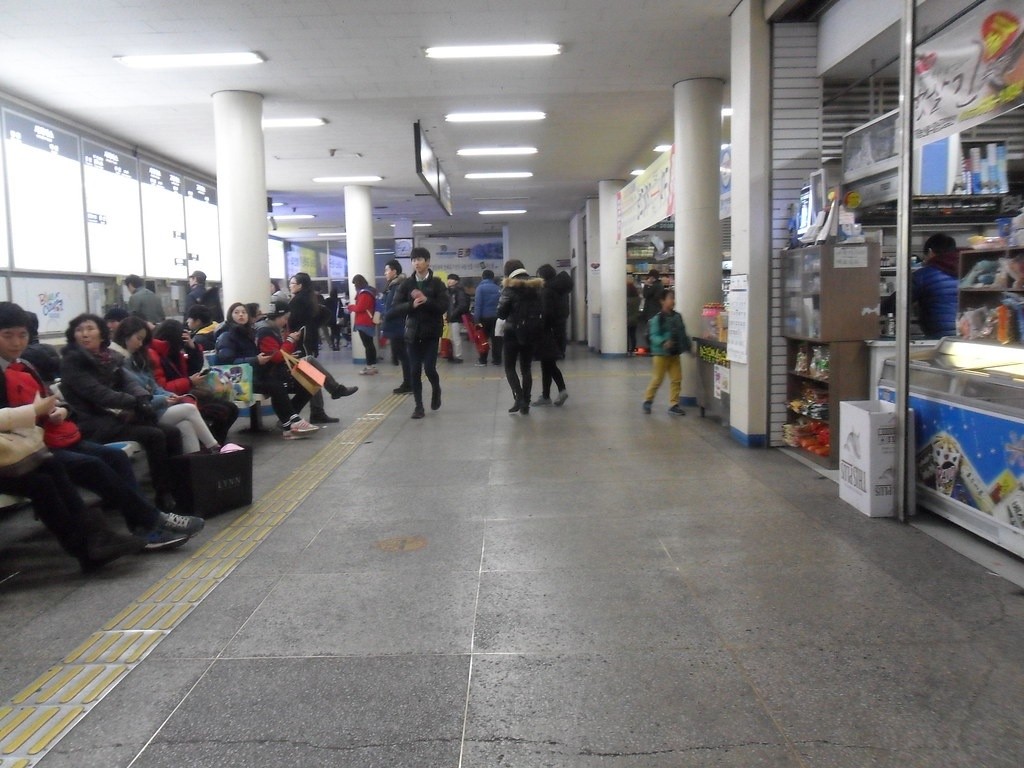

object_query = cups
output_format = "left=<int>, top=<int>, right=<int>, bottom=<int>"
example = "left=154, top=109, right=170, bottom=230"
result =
left=995, top=217, right=1010, bottom=236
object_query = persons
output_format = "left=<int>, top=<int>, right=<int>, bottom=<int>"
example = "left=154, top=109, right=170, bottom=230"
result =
left=532, top=264, right=573, bottom=406
left=626, top=276, right=641, bottom=351
left=643, top=269, right=665, bottom=322
left=391, top=248, right=450, bottom=418
left=287, top=273, right=319, bottom=357
left=327, top=289, right=345, bottom=351
left=0, top=300, right=221, bottom=564
left=881, top=234, right=959, bottom=340
left=214, top=303, right=359, bottom=440
left=382, top=260, right=412, bottom=394
left=643, top=290, right=691, bottom=416
left=314, top=286, right=336, bottom=348
left=186, top=305, right=222, bottom=351
left=446, top=273, right=470, bottom=364
left=183, top=270, right=206, bottom=327
left=348, top=275, right=379, bottom=375
left=270, top=279, right=289, bottom=312
left=497, top=260, right=545, bottom=415
left=473, top=270, right=502, bottom=367
left=125, top=274, right=166, bottom=324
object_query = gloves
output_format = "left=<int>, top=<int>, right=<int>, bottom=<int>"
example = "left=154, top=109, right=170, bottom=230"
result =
left=135, top=395, right=157, bottom=424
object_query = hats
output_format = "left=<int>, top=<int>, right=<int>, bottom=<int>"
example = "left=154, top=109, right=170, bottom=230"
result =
left=262, top=301, right=289, bottom=315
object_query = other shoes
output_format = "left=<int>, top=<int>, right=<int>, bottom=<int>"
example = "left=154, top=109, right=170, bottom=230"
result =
left=154, top=494, right=178, bottom=510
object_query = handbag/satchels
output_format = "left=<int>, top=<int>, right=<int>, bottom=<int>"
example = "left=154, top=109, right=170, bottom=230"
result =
left=181, top=442, right=252, bottom=518
left=440, top=323, right=452, bottom=358
left=475, top=324, right=491, bottom=352
left=494, top=318, right=505, bottom=336
left=280, top=349, right=326, bottom=396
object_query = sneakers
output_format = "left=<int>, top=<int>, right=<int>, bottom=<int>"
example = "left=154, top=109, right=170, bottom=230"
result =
left=411, top=407, right=425, bottom=417
left=554, top=390, right=569, bottom=407
left=431, top=392, right=441, bottom=410
left=643, top=401, right=651, bottom=412
left=310, top=413, right=339, bottom=424
left=394, top=384, right=414, bottom=394
left=159, top=513, right=204, bottom=537
left=531, top=395, right=553, bottom=407
left=143, top=528, right=188, bottom=551
left=668, top=405, right=685, bottom=414
left=283, top=430, right=310, bottom=439
left=359, top=365, right=378, bottom=374
left=332, top=384, right=358, bottom=398
left=290, top=419, right=319, bottom=434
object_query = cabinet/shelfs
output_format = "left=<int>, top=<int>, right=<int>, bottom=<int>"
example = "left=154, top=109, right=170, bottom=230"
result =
left=877, top=224, right=980, bottom=341
left=780, top=240, right=881, bottom=342
left=954, top=248, right=1024, bottom=348
left=626, top=241, right=674, bottom=309
left=782, top=338, right=868, bottom=468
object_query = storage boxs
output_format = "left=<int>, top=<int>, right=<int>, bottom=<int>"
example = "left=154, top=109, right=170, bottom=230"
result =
left=211, top=363, right=253, bottom=402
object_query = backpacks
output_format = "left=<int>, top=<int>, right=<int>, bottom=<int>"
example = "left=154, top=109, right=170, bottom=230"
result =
left=358, top=288, right=384, bottom=324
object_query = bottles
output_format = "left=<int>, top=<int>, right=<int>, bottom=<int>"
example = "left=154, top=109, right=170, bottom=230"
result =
left=885, top=313, right=895, bottom=335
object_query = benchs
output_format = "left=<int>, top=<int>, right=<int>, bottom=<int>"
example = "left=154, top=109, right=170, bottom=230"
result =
left=0, top=352, right=289, bottom=534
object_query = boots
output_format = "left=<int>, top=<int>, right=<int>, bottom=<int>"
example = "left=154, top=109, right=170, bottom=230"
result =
left=72, top=506, right=150, bottom=560
left=521, top=394, right=531, bottom=413
left=49, top=520, right=120, bottom=569
left=509, top=389, right=526, bottom=412
